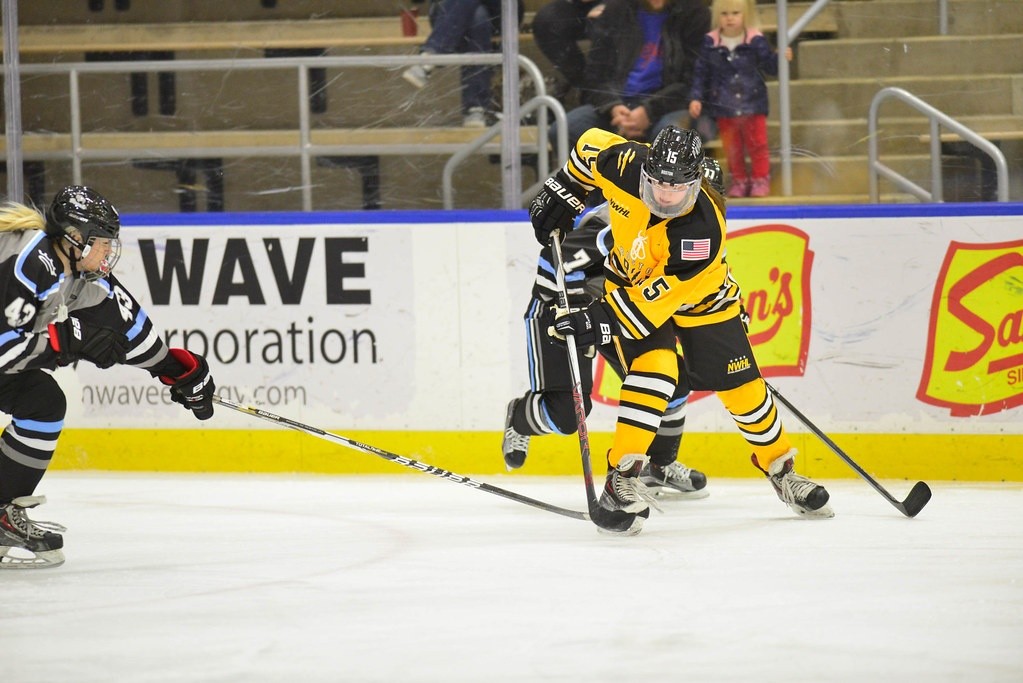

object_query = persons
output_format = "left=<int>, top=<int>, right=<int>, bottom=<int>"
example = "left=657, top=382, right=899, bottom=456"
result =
left=529, top=124, right=835, bottom=537
left=501, top=199, right=710, bottom=501
left=0, top=185, right=216, bottom=570
left=403, top=0, right=524, bottom=127
left=533, top=0, right=711, bottom=158
left=689, top=0, right=793, bottom=198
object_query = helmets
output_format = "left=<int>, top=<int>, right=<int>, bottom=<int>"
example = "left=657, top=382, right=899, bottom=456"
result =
left=638, top=126, right=705, bottom=186
left=699, top=157, right=725, bottom=195
left=45, top=184, right=122, bottom=282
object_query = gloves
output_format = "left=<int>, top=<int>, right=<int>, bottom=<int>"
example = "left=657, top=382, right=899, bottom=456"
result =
left=163, top=348, right=216, bottom=421
left=528, top=168, right=589, bottom=248
left=49, top=317, right=130, bottom=370
left=549, top=299, right=622, bottom=359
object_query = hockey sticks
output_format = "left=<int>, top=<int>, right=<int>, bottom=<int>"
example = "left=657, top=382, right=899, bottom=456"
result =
left=548, top=227, right=657, bottom=537
left=760, top=376, right=935, bottom=521
left=210, top=393, right=651, bottom=539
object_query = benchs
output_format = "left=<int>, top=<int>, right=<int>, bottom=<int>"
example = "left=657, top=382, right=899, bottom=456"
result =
left=0, top=14, right=553, bottom=208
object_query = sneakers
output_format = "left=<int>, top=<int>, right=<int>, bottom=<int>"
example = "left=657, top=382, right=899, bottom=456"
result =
left=500, top=390, right=540, bottom=472
left=404, top=50, right=436, bottom=88
left=461, top=105, right=485, bottom=126
left=751, top=448, right=834, bottom=519
left=638, top=456, right=710, bottom=499
left=0, top=495, right=69, bottom=569
left=750, top=175, right=770, bottom=197
left=596, top=448, right=649, bottom=534
left=727, top=178, right=748, bottom=197
